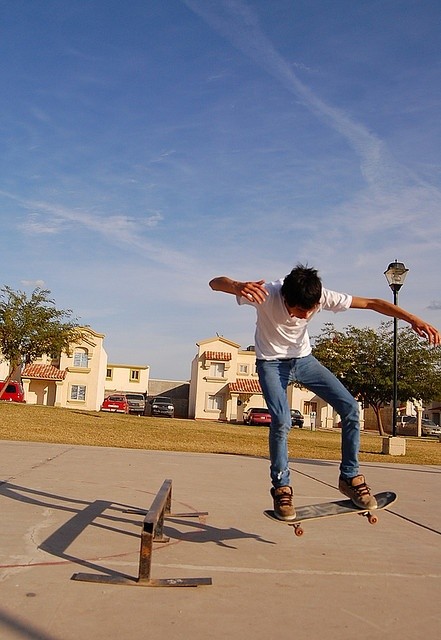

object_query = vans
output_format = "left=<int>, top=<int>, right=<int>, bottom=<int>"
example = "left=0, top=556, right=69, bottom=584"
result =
left=395, top=415, right=417, bottom=434
left=125, top=394, right=148, bottom=416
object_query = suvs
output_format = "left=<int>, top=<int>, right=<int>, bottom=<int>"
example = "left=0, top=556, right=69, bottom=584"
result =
left=151, top=397, right=174, bottom=417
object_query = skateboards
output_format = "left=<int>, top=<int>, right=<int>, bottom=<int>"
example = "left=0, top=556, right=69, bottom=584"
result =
left=263, top=491, right=398, bottom=537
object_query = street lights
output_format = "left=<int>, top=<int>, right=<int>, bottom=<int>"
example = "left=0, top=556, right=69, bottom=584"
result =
left=383, top=260, right=409, bottom=437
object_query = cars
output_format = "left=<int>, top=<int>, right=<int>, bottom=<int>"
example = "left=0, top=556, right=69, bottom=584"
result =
left=404, top=418, right=441, bottom=436
left=290, top=409, right=304, bottom=428
left=242, top=408, right=272, bottom=427
left=101, top=395, right=129, bottom=414
left=0, top=380, right=24, bottom=402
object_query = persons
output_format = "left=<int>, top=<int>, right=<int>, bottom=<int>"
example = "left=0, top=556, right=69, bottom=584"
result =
left=209, top=262, right=441, bottom=521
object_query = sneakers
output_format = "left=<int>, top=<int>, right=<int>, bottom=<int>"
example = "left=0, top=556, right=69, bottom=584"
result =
left=269, top=485, right=297, bottom=519
left=339, top=472, right=377, bottom=510
